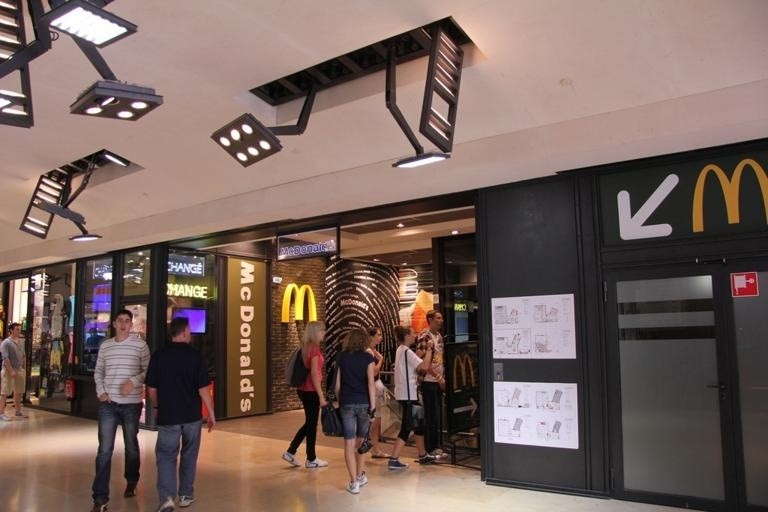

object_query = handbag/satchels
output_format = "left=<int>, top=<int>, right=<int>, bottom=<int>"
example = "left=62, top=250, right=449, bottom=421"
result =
left=410, top=405, right=425, bottom=431
left=321, top=405, right=343, bottom=437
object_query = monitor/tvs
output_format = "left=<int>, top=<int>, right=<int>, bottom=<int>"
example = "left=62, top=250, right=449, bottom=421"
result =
left=171, top=307, right=207, bottom=335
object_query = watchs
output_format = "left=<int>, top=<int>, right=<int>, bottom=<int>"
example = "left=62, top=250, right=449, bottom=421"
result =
left=428, top=347, right=433, bottom=350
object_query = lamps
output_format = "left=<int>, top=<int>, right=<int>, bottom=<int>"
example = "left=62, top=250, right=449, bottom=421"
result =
left=210, top=85, right=317, bottom=168
left=60, top=169, right=103, bottom=242
left=40, top=0, right=139, bottom=49
left=385, top=45, right=451, bottom=168
left=46, top=0, right=164, bottom=121
left=102, top=150, right=131, bottom=168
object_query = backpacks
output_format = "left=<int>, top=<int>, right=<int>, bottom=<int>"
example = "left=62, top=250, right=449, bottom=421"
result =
left=284, top=345, right=309, bottom=387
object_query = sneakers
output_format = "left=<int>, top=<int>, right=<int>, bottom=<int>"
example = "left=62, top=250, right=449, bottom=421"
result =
left=0, top=414, right=12, bottom=421
left=91, top=485, right=196, bottom=511
left=282, top=450, right=442, bottom=494
left=15, top=411, right=28, bottom=419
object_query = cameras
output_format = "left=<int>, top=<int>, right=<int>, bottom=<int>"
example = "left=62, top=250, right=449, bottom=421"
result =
left=358, top=440, right=373, bottom=454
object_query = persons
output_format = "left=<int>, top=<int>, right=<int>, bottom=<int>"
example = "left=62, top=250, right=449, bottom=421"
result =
left=331, top=328, right=376, bottom=494
left=387, top=324, right=434, bottom=470
left=0, top=323, right=106, bottom=422
left=144, top=315, right=218, bottom=511
left=416, top=309, right=449, bottom=460
left=281, top=320, right=328, bottom=469
left=364, top=327, right=392, bottom=459
left=90, top=309, right=150, bottom=512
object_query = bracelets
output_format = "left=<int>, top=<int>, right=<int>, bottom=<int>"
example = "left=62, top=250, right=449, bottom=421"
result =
left=152, top=405, right=159, bottom=409
left=435, top=373, right=442, bottom=381
